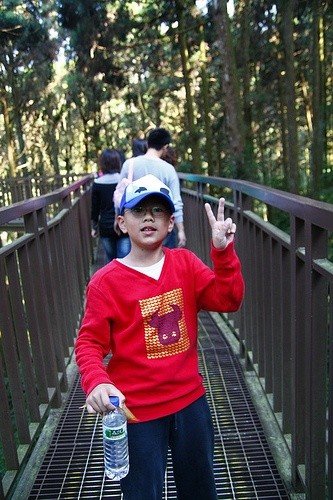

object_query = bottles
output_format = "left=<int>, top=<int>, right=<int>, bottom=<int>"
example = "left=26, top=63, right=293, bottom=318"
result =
left=102, top=396, right=130, bottom=481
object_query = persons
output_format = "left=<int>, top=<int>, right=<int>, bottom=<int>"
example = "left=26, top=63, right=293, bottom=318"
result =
left=75, top=174, right=244, bottom=500
left=91, top=128, right=186, bottom=266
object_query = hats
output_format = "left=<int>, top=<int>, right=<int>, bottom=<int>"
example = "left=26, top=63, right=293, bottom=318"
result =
left=119, top=174, right=176, bottom=215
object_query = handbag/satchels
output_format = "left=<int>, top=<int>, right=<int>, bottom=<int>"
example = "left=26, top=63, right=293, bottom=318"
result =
left=114, top=157, right=134, bottom=211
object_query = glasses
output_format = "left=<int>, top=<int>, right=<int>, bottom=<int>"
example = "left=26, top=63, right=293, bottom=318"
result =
left=124, top=205, right=166, bottom=217
